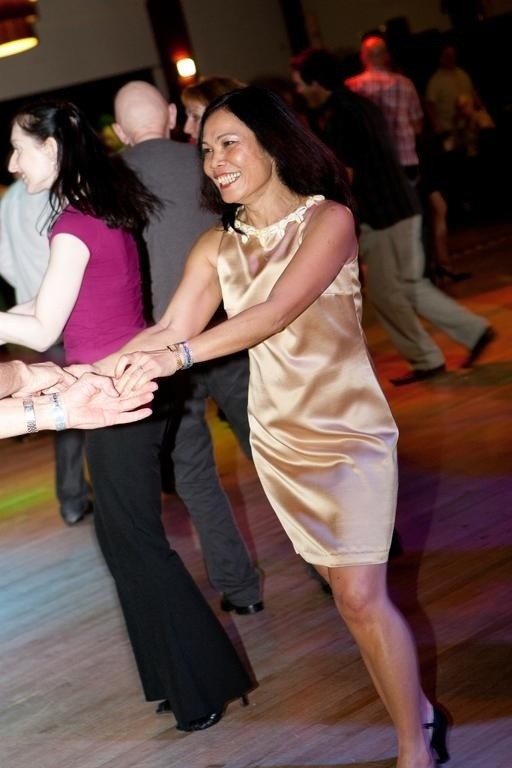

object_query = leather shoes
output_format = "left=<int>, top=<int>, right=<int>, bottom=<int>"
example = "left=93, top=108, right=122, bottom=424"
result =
left=385, top=357, right=447, bottom=389
left=220, top=596, right=262, bottom=616
left=455, top=323, right=499, bottom=373
left=62, top=499, right=95, bottom=526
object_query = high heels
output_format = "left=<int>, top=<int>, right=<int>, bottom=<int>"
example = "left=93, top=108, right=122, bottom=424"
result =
left=433, top=261, right=475, bottom=289
left=156, top=699, right=172, bottom=715
left=417, top=684, right=452, bottom=767
left=173, top=692, right=251, bottom=733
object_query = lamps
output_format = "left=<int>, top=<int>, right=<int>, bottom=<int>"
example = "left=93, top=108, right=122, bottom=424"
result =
left=0, top=1, right=41, bottom=57
